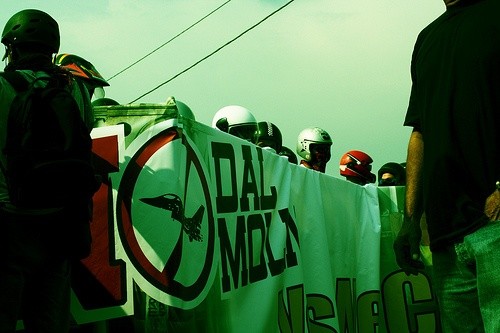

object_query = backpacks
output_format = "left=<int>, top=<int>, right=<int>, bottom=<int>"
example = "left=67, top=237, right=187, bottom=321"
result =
left=1, top=67, right=96, bottom=209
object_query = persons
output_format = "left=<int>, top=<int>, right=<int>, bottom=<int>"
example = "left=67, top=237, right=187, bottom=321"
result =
left=378, top=163, right=407, bottom=187
left=256, top=121, right=283, bottom=155
left=52, top=54, right=110, bottom=102
left=394, top=0, right=500, bottom=333
left=212, top=105, right=258, bottom=145
left=340, top=149, right=376, bottom=186
left=0, top=10, right=94, bottom=332
left=297, top=126, right=333, bottom=173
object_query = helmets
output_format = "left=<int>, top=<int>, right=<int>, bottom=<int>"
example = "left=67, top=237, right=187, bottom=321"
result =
left=211, top=105, right=257, bottom=142
left=1, top=9, right=60, bottom=53
left=166, top=95, right=195, bottom=120
left=296, top=127, right=333, bottom=163
left=253, top=121, right=282, bottom=154
left=279, top=146, right=298, bottom=165
left=51, top=53, right=110, bottom=87
left=339, top=149, right=376, bottom=183
left=91, top=97, right=119, bottom=106
left=378, top=162, right=406, bottom=186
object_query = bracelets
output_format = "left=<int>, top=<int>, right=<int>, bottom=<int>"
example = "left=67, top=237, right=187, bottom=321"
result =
left=496, top=181, right=500, bottom=191
